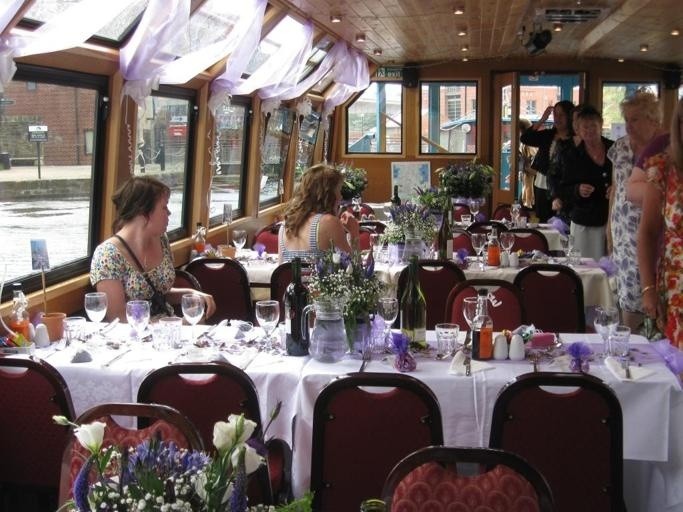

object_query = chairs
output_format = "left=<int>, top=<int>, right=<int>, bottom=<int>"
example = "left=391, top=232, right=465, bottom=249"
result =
left=256, top=220, right=279, bottom=253
left=502, top=229, right=549, bottom=256
left=340, top=205, right=374, bottom=219
left=310, top=373, right=442, bottom=512
left=514, top=264, right=586, bottom=333
left=185, top=258, right=252, bottom=325
left=467, top=222, right=505, bottom=241
left=443, top=280, right=524, bottom=332
left=392, top=260, right=465, bottom=330
left=453, top=203, right=473, bottom=221
left=134, top=361, right=271, bottom=512
left=381, top=446, right=556, bottom=512
left=434, top=229, right=476, bottom=255
left=59, top=404, right=200, bottom=507
left=494, top=204, right=529, bottom=223
left=173, top=270, right=200, bottom=317
left=0, top=353, right=76, bottom=511
left=359, top=222, right=384, bottom=250
left=271, top=262, right=314, bottom=326
left=482, top=372, right=624, bottom=511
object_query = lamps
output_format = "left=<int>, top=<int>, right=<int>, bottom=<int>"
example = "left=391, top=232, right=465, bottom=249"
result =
left=330, top=16, right=341, bottom=22
left=554, top=23, right=562, bottom=31
left=639, top=44, right=647, bottom=52
left=356, top=36, right=365, bottom=41
left=374, top=50, right=382, bottom=55
left=457, top=30, right=467, bottom=36
left=519, top=24, right=551, bottom=56
left=461, top=45, right=469, bottom=51
left=454, top=7, right=464, bottom=14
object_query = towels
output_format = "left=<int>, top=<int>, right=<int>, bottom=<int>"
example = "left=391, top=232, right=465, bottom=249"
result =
left=605, top=357, right=654, bottom=381
left=448, top=351, right=494, bottom=375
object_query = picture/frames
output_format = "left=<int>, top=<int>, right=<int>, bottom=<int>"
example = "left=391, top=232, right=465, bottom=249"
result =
left=391, top=161, right=431, bottom=206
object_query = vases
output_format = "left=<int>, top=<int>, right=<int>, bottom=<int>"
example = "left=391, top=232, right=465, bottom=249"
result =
left=451, top=198, right=485, bottom=214
left=341, top=199, right=352, bottom=205
left=399, top=241, right=425, bottom=259
left=425, top=208, right=441, bottom=227
left=345, top=310, right=374, bottom=360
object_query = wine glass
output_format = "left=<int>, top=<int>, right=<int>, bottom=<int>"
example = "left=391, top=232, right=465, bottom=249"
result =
left=499, top=230, right=515, bottom=264
left=377, top=298, right=400, bottom=337
left=85, top=292, right=109, bottom=338
left=126, top=299, right=151, bottom=346
left=559, top=233, right=575, bottom=261
left=255, top=300, right=282, bottom=336
left=594, top=306, right=620, bottom=355
left=382, top=206, right=392, bottom=223
left=510, top=204, right=522, bottom=224
left=231, top=230, right=248, bottom=255
left=368, top=233, right=385, bottom=257
left=462, top=297, right=481, bottom=349
left=470, top=234, right=489, bottom=264
left=181, top=294, right=206, bottom=343
left=469, top=201, right=481, bottom=223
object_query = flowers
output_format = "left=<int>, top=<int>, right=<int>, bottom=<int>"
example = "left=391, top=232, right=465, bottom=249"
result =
left=434, top=156, right=495, bottom=197
left=309, top=242, right=381, bottom=351
left=51, top=413, right=315, bottom=512
left=416, top=186, right=447, bottom=208
left=386, top=203, right=438, bottom=245
left=337, top=164, right=367, bottom=199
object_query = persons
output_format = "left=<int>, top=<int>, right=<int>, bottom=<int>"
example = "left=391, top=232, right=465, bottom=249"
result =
left=274, top=162, right=362, bottom=272
left=545, top=103, right=594, bottom=225
left=88, top=173, right=218, bottom=325
left=625, top=131, right=671, bottom=203
left=504, top=117, right=537, bottom=209
left=520, top=99, right=574, bottom=223
left=552, top=108, right=616, bottom=261
left=603, top=89, right=665, bottom=330
left=637, top=95, right=683, bottom=352
left=157, top=137, right=165, bottom=171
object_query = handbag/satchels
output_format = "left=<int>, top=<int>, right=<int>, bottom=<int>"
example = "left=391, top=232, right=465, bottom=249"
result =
left=531, top=148, right=549, bottom=175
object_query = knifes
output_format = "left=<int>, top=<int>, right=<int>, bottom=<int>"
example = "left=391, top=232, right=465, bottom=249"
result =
left=462, top=356, right=472, bottom=377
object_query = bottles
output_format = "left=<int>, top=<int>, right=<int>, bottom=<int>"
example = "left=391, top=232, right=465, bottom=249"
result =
left=390, top=184, right=402, bottom=206
left=399, top=256, right=429, bottom=350
left=470, top=288, right=493, bottom=361
left=282, top=257, right=311, bottom=356
left=193, top=222, right=206, bottom=253
left=437, top=213, right=454, bottom=260
left=10, top=283, right=29, bottom=342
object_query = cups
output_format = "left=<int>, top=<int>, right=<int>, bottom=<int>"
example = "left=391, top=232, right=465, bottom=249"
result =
left=41, top=312, right=67, bottom=341
left=460, top=215, right=472, bottom=224
left=568, top=247, right=581, bottom=264
left=435, top=322, right=459, bottom=358
left=63, top=316, right=87, bottom=346
left=609, top=325, right=632, bottom=358
left=153, top=316, right=182, bottom=350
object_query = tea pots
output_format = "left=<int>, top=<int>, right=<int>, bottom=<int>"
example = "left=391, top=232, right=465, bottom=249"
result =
left=298, top=300, right=351, bottom=365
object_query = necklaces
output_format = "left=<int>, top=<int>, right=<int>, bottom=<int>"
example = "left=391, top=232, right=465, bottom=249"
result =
left=585, top=143, right=602, bottom=161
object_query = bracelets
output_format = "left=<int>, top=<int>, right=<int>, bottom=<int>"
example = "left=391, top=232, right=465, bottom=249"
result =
left=641, top=284, right=655, bottom=294
